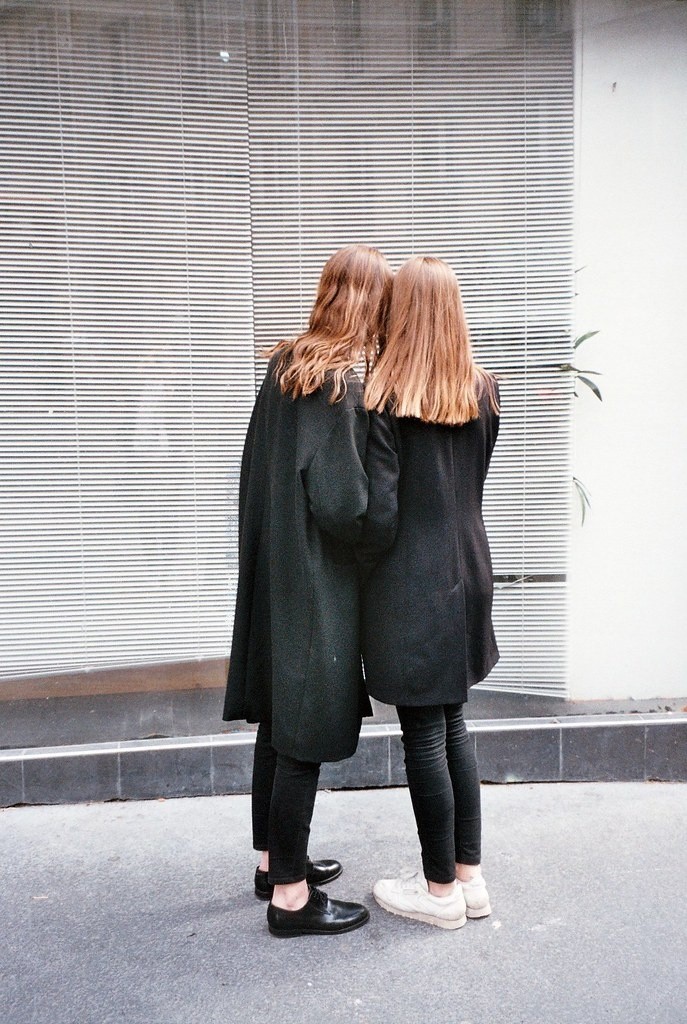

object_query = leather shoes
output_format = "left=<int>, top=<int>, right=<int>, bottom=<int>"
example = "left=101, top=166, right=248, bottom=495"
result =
left=254, top=854, right=344, bottom=901
left=266, top=884, right=369, bottom=938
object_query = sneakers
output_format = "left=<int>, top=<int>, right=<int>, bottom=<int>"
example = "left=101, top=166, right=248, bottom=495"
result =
left=458, top=873, right=492, bottom=919
left=372, top=869, right=464, bottom=930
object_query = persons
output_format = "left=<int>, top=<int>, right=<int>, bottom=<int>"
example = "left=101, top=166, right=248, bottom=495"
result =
left=360, top=256, right=501, bottom=928
left=223, top=244, right=398, bottom=939
